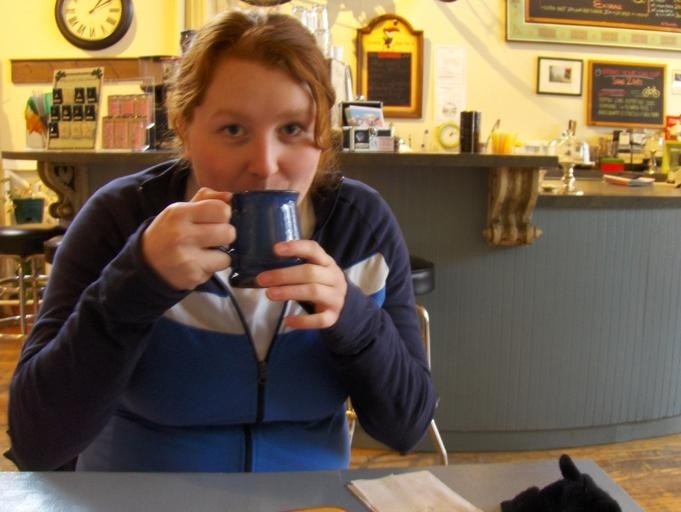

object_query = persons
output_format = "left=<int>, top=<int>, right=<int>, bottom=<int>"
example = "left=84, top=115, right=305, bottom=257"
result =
left=8, top=9, right=436, bottom=474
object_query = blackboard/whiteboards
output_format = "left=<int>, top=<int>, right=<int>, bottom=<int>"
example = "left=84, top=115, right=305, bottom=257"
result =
left=524, top=0, right=681, bottom=34
left=586, top=59, right=668, bottom=128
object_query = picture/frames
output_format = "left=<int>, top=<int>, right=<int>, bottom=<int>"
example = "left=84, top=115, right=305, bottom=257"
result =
left=536, top=55, right=585, bottom=98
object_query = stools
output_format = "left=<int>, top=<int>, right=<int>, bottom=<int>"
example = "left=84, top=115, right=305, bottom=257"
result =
left=336, top=251, right=450, bottom=471
left=1, top=219, right=67, bottom=342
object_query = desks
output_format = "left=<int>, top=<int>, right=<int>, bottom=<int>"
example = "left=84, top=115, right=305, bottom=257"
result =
left=0, top=452, right=651, bottom=512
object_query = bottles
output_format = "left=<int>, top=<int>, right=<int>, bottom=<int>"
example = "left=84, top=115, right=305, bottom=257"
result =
left=459, top=110, right=483, bottom=153
left=100, top=93, right=154, bottom=150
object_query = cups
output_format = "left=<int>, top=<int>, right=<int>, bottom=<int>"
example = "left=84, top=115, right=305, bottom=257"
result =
left=209, top=188, right=308, bottom=291
left=289, top=4, right=332, bottom=57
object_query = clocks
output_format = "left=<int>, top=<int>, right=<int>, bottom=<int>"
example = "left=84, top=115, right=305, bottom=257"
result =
left=53, top=0, right=134, bottom=52
